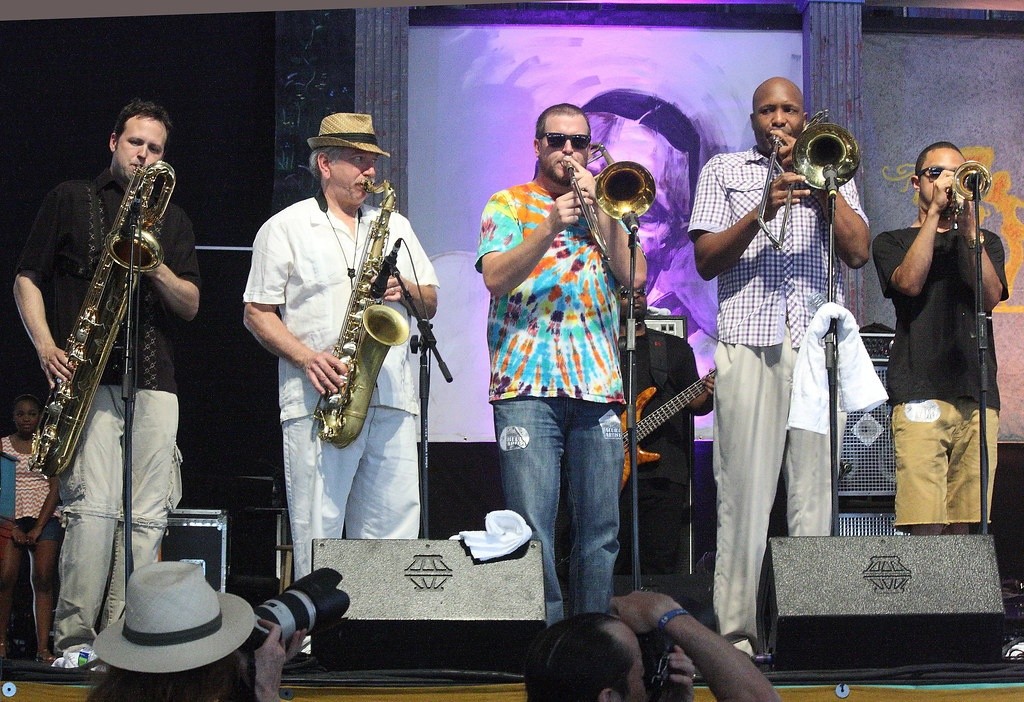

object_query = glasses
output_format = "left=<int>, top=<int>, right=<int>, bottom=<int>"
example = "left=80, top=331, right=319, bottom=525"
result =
left=918, top=166, right=945, bottom=178
left=538, top=132, right=591, bottom=149
left=618, top=286, right=648, bottom=299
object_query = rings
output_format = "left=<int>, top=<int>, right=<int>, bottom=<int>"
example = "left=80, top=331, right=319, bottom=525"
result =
left=392, top=287, right=395, bottom=295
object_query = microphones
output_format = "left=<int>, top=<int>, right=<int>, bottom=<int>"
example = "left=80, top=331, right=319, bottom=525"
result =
left=370, top=238, right=401, bottom=299
left=120, top=189, right=143, bottom=241
left=963, top=172, right=982, bottom=191
left=823, top=163, right=838, bottom=199
left=620, top=211, right=641, bottom=233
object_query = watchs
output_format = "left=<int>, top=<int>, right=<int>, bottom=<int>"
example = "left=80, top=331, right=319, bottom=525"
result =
left=966, top=231, right=985, bottom=248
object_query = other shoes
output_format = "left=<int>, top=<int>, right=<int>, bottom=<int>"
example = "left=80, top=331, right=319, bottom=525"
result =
left=62, top=647, right=109, bottom=673
left=36, top=649, right=58, bottom=663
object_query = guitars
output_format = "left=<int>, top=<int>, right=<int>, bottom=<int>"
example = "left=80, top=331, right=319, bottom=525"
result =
left=619, top=368, right=717, bottom=493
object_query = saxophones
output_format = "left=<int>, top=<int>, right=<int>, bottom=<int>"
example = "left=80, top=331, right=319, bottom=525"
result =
left=311, top=177, right=411, bottom=450
left=27, top=158, right=179, bottom=479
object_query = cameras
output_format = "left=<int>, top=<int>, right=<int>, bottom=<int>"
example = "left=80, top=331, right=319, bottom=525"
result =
left=637, top=630, right=674, bottom=690
left=236, top=568, right=350, bottom=654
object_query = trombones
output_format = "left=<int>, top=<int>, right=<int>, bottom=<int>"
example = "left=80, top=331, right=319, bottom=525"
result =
left=560, top=142, right=657, bottom=263
left=755, top=107, right=862, bottom=251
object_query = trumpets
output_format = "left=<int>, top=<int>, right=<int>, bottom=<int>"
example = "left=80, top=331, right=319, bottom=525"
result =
left=946, top=158, right=993, bottom=232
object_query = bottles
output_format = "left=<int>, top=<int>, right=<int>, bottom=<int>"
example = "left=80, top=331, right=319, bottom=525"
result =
left=64, top=648, right=112, bottom=673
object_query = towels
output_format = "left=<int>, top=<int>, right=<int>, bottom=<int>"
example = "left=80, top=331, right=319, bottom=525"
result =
left=448, top=509, right=533, bottom=562
left=787, top=302, right=890, bottom=435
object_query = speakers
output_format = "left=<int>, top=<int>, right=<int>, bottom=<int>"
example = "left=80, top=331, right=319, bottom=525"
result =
left=757, top=326, right=1005, bottom=670
left=310, top=538, right=547, bottom=670
left=612, top=572, right=715, bottom=636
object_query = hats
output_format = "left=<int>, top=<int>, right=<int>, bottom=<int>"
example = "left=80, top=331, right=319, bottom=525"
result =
left=93, top=561, right=255, bottom=674
left=307, top=112, right=391, bottom=158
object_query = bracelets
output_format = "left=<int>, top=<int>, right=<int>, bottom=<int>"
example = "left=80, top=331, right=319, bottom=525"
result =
left=658, top=608, right=688, bottom=633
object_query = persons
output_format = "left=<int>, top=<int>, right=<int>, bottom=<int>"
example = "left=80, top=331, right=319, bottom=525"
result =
left=11, top=98, right=202, bottom=678
left=89, top=559, right=307, bottom=702
left=522, top=590, right=782, bottom=702
left=617, top=278, right=715, bottom=587
left=243, top=111, right=438, bottom=658
left=686, top=77, right=871, bottom=660
left=872, top=142, right=1009, bottom=535
left=473, top=105, right=647, bottom=630
left=0, top=388, right=59, bottom=664
